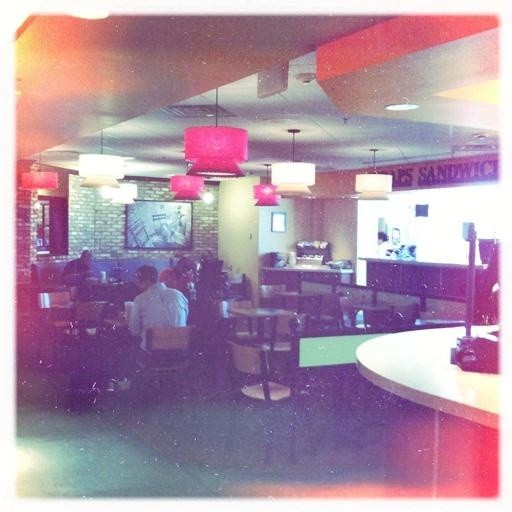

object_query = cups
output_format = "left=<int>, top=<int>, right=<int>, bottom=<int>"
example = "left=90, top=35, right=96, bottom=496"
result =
left=39, top=292, right=51, bottom=309
left=220, top=301, right=228, bottom=318
left=125, top=302, right=134, bottom=320
left=289, top=252, right=296, bottom=265
left=100, top=271, right=107, bottom=283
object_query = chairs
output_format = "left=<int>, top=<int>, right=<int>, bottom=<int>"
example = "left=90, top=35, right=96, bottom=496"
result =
left=35, top=274, right=419, bottom=408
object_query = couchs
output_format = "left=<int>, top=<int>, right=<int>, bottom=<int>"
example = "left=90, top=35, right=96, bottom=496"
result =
left=31, top=258, right=180, bottom=294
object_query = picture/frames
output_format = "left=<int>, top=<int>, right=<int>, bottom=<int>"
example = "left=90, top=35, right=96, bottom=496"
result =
left=271, top=211, right=286, bottom=233
left=125, top=199, right=193, bottom=249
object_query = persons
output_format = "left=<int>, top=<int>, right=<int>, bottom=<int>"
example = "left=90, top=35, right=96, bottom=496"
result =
left=120, top=263, right=189, bottom=385
left=377, top=231, right=392, bottom=259
left=190, top=256, right=227, bottom=359
left=159, top=258, right=196, bottom=300
left=62, top=249, right=96, bottom=294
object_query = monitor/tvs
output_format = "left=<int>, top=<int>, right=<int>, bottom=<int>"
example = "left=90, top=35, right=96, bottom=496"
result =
left=478, top=239, right=500, bottom=265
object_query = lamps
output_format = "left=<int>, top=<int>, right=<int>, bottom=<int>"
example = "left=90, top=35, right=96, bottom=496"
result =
left=101, top=184, right=139, bottom=206
left=183, top=126, right=251, bottom=181
left=169, top=175, right=205, bottom=201
left=21, top=172, right=59, bottom=191
left=354, top=174, right=393, bottom=200
left=251, top=184, right=279, bottom=205
left=77, top=154, right=126, bottom=190
left=271, top=162, right=317, bottom=199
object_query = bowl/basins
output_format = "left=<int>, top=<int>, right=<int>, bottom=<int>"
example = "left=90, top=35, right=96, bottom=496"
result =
left=86, top=327, right=96, bottom=335
